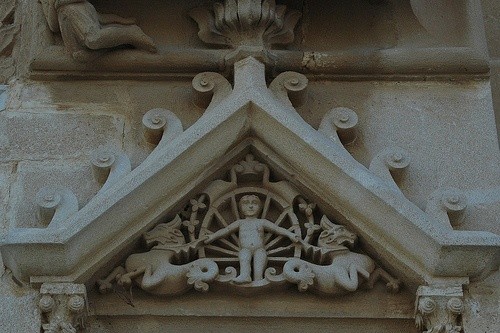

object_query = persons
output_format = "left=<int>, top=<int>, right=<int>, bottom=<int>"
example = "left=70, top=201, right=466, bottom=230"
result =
left=39, top=0, right=156, bottom=64
left=205, top=194, right=301, bottom=284
